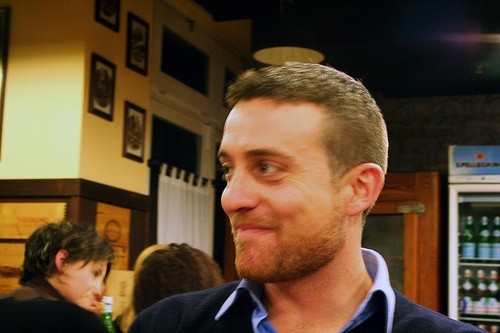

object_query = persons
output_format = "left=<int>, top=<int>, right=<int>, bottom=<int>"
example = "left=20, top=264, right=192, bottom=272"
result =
left=124, top=62, right=490, bottom=333
left=0, top=221, right=114, bottom=333
left=130, top=243, right=223, bottom=317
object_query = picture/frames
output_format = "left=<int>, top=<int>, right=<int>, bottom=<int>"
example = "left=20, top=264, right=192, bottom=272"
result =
left=160, top=26, right=210, bottom=98
left=125, top=11, right=149, bottom=76
left=93, top=0, right=122, bottom=33
left=223, top=67, right=236, bottom=108
left=88, top=52, right=117, bottom=122
left=123, top=100, right=147, bottom=163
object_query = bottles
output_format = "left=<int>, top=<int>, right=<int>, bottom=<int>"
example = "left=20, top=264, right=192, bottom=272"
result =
left=461, top=216, right=476, bottom=258
left=460, top=268, right=475, bottom=312
left=97, top=295, right=119, bottom=333
left=488, top=270, right=500, bottom=312
left=491, top=325, right=498, bottom=333
left=477, top=216, right=491, bottom=258
left=490, top=215, right=500, bottom=258
left=475, top=269, right=489, bottom=312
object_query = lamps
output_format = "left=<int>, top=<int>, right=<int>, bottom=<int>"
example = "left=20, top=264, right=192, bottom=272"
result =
left=251, top=0, right=326, bottom=64
